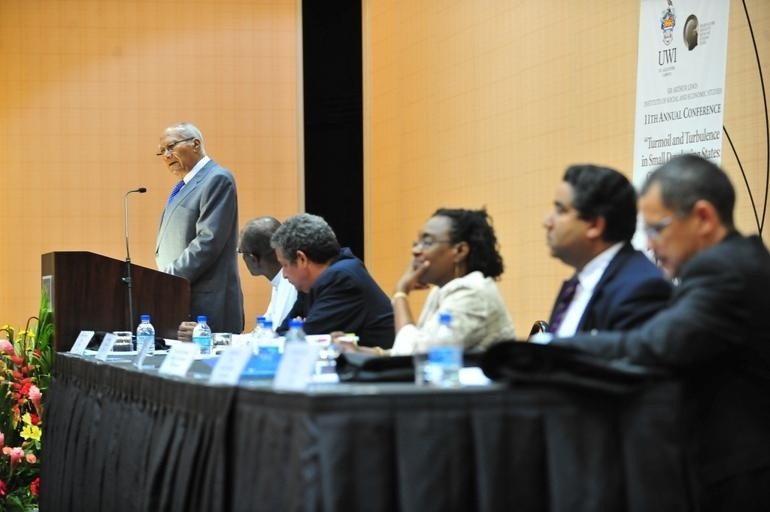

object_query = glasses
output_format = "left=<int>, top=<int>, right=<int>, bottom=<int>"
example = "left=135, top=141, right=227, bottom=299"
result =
left=155, top=137, right=194, bottom=155
left=237, top=248, right=255, bottom=256
left=643, top=211, right=687, bottom=240
left=412, top=238, right=453, bottom=246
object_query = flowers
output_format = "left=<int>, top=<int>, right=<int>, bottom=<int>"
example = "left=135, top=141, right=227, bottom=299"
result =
left=0, top=284, right=55, bottom=512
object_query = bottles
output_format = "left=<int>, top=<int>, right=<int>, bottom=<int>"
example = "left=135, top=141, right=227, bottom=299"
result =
left=258, top=321, right=279, bottom=375
left=136, top=315, right=155, bottom=357
left=284, top=319, right=306, bottom=344
left=425, top=314, right=464, bottom=383
left=192, top=316, right=213, bottom=359
left=252, top=317, right=266, bottom=355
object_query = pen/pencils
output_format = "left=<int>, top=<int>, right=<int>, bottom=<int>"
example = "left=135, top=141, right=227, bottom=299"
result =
left=338, top=336, right=355, bottom=343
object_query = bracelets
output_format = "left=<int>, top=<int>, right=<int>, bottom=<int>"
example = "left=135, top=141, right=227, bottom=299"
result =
left=391, top=290, right=407, bottom=306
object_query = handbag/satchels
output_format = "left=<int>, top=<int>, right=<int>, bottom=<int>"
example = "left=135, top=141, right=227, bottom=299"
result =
left=480, top=340, right=675, bottom=398
left=334, top=352, right=486, bottom=383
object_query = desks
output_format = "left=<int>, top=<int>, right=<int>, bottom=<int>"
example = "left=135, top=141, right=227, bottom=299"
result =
left=39, top=342, right=708, bottom=512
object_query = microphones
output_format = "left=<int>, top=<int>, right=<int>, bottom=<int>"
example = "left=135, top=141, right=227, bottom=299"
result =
left=124, top=187, right=147, bottom=259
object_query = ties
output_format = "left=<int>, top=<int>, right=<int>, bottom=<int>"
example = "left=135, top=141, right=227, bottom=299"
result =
left=167, top=180, right=185, bottom=208
left=547, top=275, right=580, bottom=332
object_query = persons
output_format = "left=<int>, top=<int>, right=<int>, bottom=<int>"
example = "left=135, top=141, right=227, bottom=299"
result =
left=270, top=212, right=394, bottom=349
left=389, top=201, right=516, bottom=361
left=151, top=120, right=249, bottom=336
left=473, top=151, right=768, bottom=511
left=237, top=212, right=298, bottom=333
left=517, top=158, right=680, bottom=345
